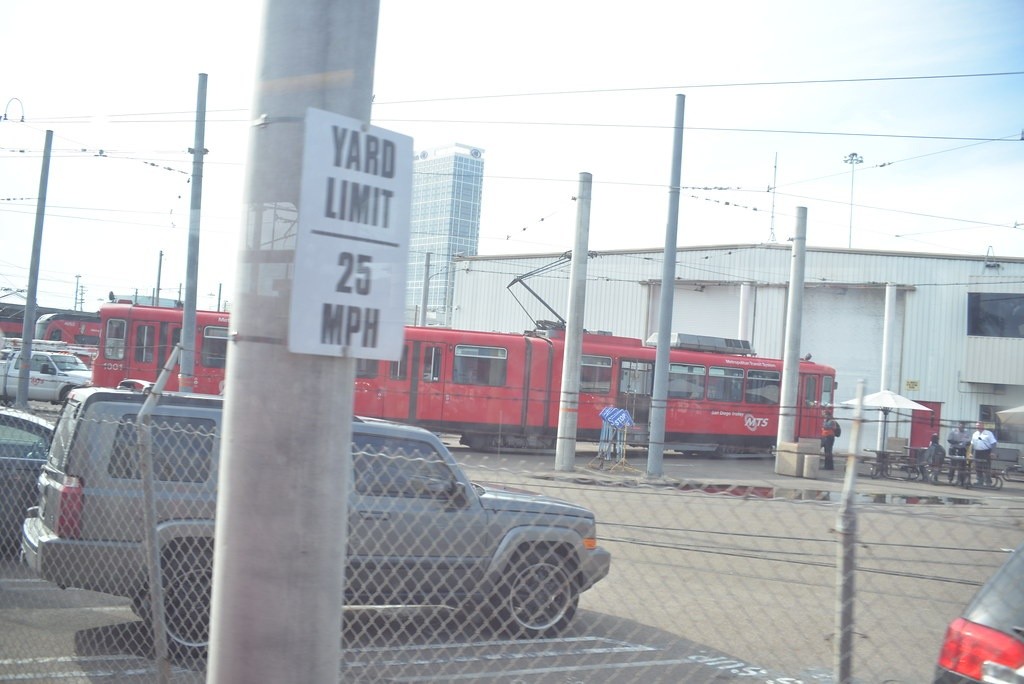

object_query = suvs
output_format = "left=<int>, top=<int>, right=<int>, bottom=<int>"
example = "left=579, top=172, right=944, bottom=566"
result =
left=19, top=379, right=612, bottom=670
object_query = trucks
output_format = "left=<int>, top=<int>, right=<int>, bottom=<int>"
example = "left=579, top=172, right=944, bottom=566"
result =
left=0, top=337, right=93, bottom=405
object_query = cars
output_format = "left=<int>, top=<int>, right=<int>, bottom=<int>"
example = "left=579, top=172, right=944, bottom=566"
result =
left=0, top=406, right=55, bottom=558
left=932, top=546, right=1024, bottom=684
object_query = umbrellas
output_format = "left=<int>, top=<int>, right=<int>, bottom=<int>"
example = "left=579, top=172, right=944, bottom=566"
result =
left=994, top=406, right=1024, bottom=424
left=840, top=390, right=932, bottom=450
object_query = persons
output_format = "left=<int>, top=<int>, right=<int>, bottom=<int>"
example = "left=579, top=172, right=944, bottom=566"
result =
left=970, top=421, right=996, bottom=486
left=948, top=422, right=972, bottom=483
left=822, top=410, right=836, bottom=469
left=920, top=435, right=946, bottom=481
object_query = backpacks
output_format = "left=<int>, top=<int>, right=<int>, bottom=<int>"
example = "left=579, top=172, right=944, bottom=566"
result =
left=827, top=419, right=841, bottom=437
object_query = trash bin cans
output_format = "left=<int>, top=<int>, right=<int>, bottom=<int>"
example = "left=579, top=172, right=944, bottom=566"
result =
left=803, top=454, right=820, bottom=479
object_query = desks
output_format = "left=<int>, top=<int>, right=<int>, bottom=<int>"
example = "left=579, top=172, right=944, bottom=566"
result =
left=863, top=448, right=903, bottom=479
left=945, top=456, right=988, bottom=490
left=903, top=446, right=929, bottom=481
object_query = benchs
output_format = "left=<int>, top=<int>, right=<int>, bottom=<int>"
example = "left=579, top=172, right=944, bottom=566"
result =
left=862, top=458, right=1004, bottom=490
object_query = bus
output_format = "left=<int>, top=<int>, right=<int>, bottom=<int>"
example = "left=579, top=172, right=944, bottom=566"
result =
left=35, top=312, right=101, bottom=370
left=92, top=299, right=838, bottom=460
